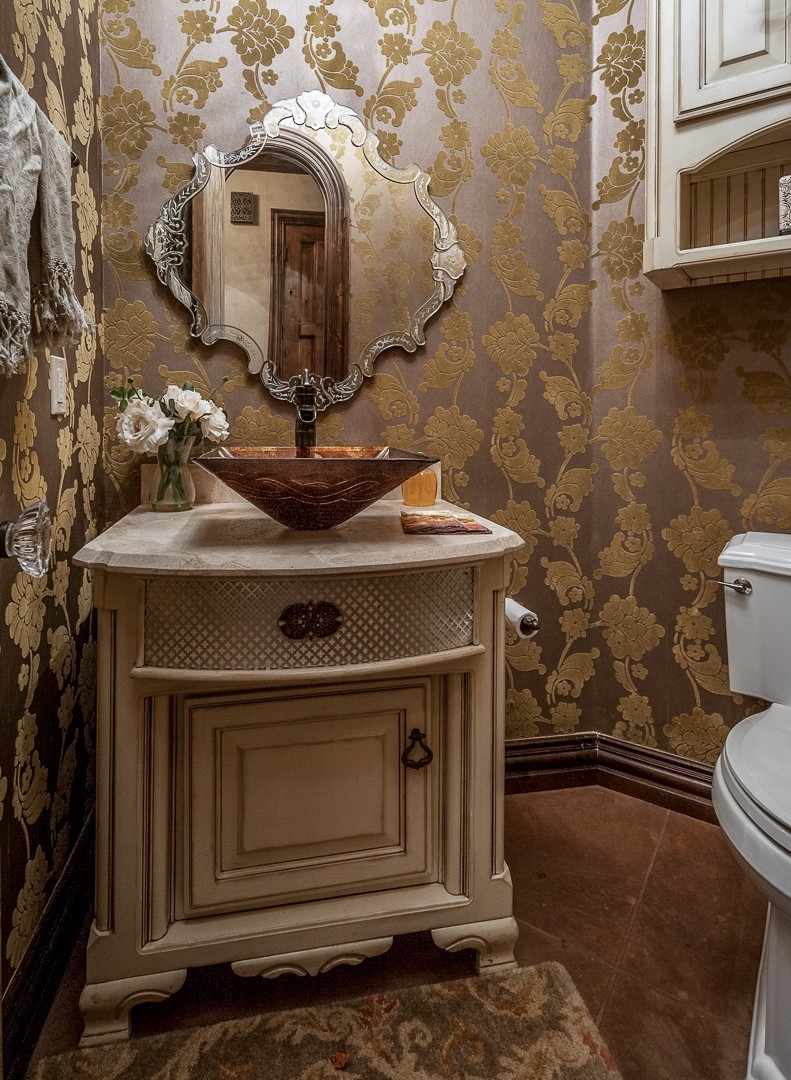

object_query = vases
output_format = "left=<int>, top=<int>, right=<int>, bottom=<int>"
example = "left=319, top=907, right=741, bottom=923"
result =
left=150, top=433, right=196, bottom=510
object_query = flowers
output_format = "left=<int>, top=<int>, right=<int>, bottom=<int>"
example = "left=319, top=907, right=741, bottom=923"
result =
left=114, top=378, right=228, bottom=510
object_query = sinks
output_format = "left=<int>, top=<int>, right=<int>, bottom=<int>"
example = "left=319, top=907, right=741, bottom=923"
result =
left=192, top=442, right=447, bottom=530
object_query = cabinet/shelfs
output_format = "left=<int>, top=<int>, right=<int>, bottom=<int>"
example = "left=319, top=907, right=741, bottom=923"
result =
left=66, top=499, right=528, bottom=1046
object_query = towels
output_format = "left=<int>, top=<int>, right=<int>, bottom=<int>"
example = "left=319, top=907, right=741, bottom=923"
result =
left=399, top=508, right=494, bottom=535
left=0, top=54, right=42, bottom=381
left=34, top=104, right=95, bottom=350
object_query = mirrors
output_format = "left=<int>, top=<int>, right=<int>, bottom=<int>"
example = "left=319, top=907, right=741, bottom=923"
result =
left=141, top=87, right=464, bottom=412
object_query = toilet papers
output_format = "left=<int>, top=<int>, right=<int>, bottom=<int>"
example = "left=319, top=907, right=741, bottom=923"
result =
left=504, top=596, right=541, bottom=641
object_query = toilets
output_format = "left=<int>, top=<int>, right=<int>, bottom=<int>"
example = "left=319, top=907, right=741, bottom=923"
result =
left=709, top=524, right=790, bottom=1080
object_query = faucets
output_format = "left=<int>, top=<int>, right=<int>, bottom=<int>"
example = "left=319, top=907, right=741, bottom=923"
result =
left=291, top=368, right=321, bottom=447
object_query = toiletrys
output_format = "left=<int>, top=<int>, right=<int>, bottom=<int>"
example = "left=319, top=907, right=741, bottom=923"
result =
left=400, top=450, right=439, bottom=507
